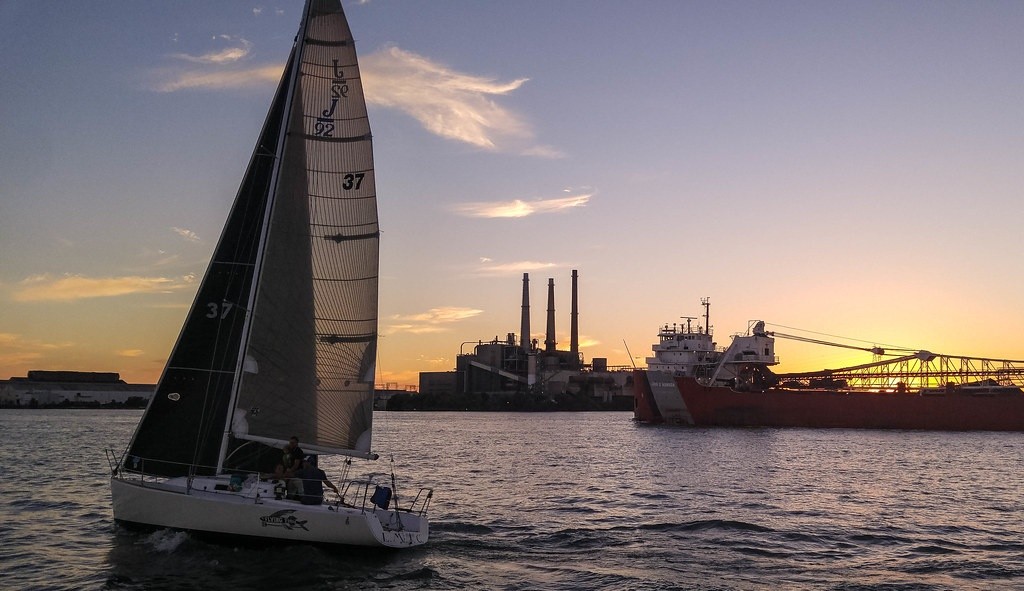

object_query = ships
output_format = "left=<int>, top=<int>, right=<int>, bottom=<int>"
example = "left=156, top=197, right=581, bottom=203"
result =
left=629, top=295, right=1024, bottom=434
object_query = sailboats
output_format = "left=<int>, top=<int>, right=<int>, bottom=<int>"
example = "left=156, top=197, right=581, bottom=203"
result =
left=99, top=0, right=438, bottom=554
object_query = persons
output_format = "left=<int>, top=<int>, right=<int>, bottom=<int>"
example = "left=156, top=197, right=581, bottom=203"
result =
left=271, top=456, right=343, bottom=506
left=285, top=436, right=305, bottom=501
left=267, top=462, right=286, bottom=500
